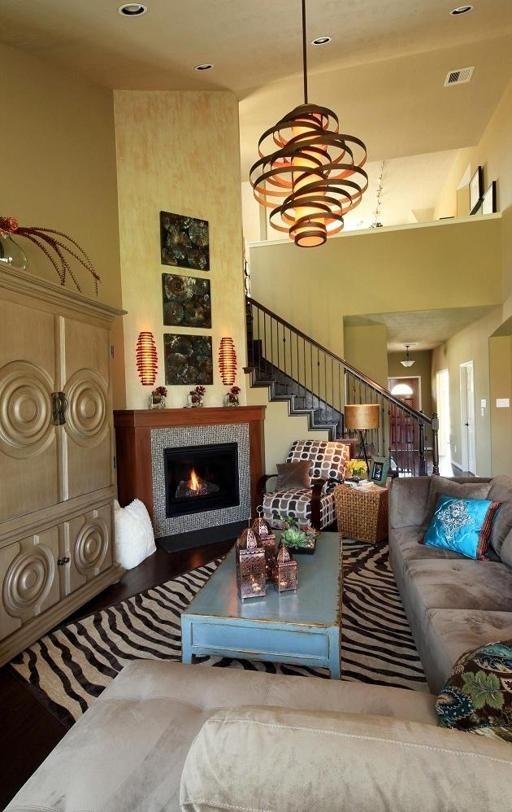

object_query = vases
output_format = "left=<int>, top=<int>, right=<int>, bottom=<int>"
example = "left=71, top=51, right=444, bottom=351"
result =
left=1, top=228, right=27, bottom=270
left=353, top=469, right=362, bottom=476
left=188, top=395, right=203, bottom=407
left=224, top=395, right=238, bottom=407
left=150, top=396, right=166, bottom=409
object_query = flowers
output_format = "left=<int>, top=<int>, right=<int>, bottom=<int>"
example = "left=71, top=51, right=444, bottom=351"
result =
left=227, top=386, right=240, bottom=405
left=190, top=385, right=205, bottom=404
left=2, top=215, right=17, bottom=238
left=345, top=458, right=368, bottom=473
left=151, top=386, right=170, bottom=402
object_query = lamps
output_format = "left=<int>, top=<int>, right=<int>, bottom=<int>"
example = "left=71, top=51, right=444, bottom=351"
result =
left=218, top=337, right=239, bottom=385
left=249, top=0, right=369, bottom=249
left=344, top=404, right=380, bottom=475
left=400, top=343, right=416, bottom=368
left=136, top=332, right=158, bottom=385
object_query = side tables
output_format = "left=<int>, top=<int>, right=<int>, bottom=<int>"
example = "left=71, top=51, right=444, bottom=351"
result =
left=334, top=482, right=389, bottom=545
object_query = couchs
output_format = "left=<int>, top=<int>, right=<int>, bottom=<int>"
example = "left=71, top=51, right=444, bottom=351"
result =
left=255, top=440, right=351, bottom=533
left=0, top=658, right=511, bottom=812
left=388, top=477, right=512, bottom=697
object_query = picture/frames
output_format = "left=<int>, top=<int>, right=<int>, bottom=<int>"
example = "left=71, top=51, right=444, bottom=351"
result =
left=481, top=180, right=496, bottom=213
left=468, top=166, right=484, bottom=215
left=369, top=455, right=390, bottom=486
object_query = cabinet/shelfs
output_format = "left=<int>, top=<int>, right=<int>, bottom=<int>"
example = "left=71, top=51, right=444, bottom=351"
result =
left=0, top=264, right=129, bottom=669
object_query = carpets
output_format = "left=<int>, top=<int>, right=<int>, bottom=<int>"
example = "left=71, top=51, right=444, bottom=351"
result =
left=4, top=533, right=431, bottom=728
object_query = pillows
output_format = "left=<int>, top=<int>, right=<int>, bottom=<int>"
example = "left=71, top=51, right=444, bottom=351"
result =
left=418, top=492, right=502, bottom=561
left=276, top=460, right=313, bottom=490
left=432, top=637, right=511, bottom=731
left=420, top=473, right=495, bottom=531
left=476, top=728, right=511, bottom=744
